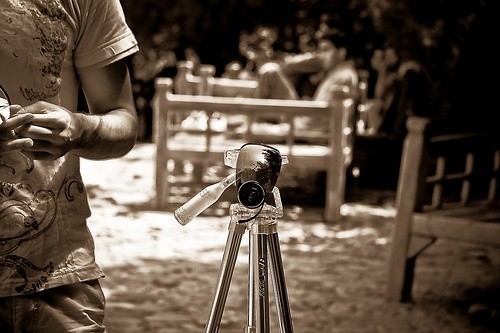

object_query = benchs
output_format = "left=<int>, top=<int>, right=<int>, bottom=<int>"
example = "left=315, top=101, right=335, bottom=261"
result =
left=152, top=78, right=355, bottom=223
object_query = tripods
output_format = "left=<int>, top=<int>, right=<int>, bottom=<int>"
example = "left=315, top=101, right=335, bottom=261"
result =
left=174, top=142, right=294, bottom=333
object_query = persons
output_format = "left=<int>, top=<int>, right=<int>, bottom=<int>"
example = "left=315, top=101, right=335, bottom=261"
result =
left=241, top=31, right=358, bottom=143
left=0, top=0, right=140, bottom=333
